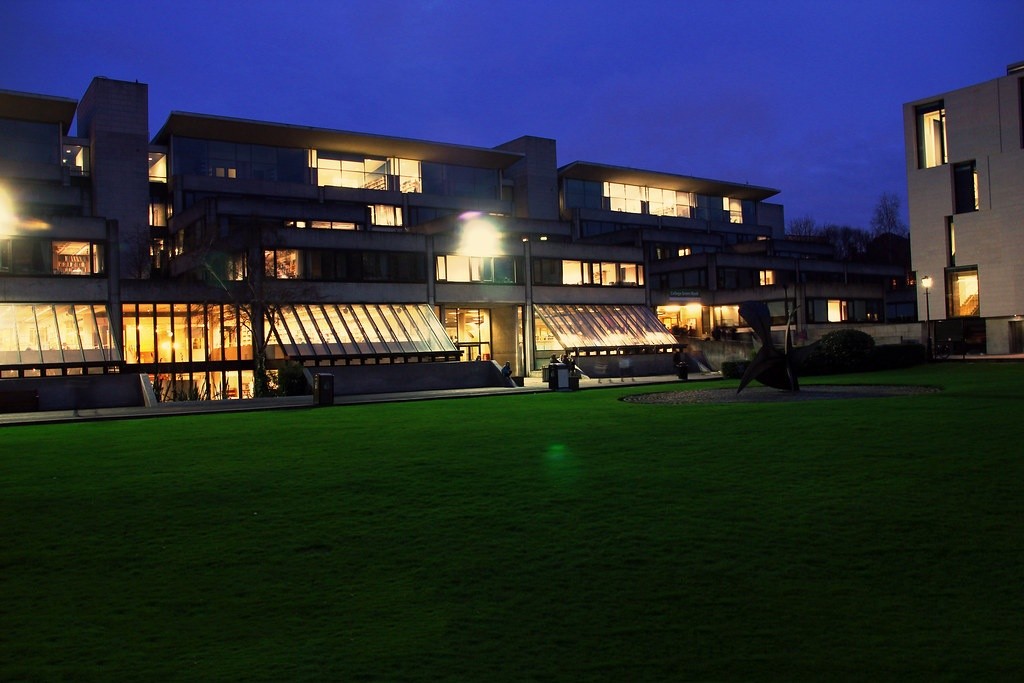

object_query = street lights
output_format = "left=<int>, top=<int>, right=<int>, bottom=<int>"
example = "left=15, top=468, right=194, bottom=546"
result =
left=922, top=276, right=934, bottom=358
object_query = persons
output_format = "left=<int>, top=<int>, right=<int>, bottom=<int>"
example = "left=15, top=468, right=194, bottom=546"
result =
left=550, top=355, right=560, bottom=363
left=501, top=361, right=512, bottom=380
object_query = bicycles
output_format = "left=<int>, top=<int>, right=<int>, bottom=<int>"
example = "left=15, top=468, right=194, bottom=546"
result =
left=930, top=343, right=951, bottom=360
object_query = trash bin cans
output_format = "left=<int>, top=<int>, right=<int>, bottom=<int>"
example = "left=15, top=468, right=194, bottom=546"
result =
left=549, top=361, right=580, bottom=389
left=312, top=372, right=334, bottom=405
left=677, top=362, right=688, bottom=379
